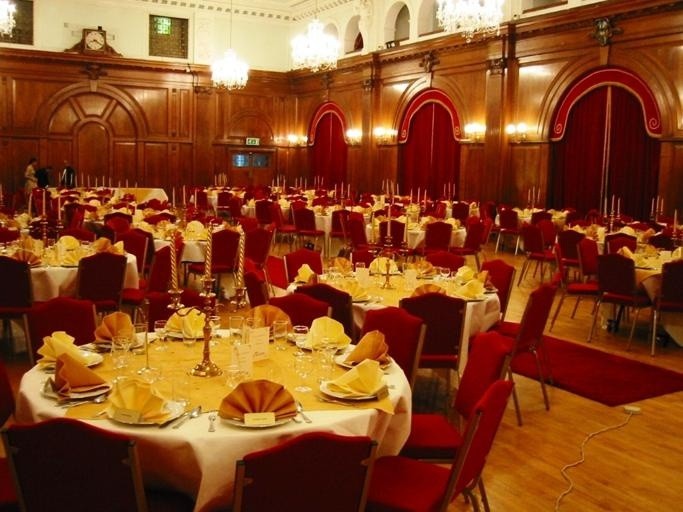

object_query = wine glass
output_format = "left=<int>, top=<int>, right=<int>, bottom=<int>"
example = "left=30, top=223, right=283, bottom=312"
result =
left=323, top=256, right=450, bottom=291
left=111, top=316, right=335, bottom=391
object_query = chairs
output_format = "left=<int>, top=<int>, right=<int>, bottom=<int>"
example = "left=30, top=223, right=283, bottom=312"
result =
left=548, top=208, right=683, bottom=357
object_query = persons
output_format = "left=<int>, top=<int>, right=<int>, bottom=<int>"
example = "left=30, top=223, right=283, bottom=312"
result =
left=60, top=160, right=76, bottom=186
left=24, top=157, right=39, bottom=202
left=34, top=165, right=53, bottom=188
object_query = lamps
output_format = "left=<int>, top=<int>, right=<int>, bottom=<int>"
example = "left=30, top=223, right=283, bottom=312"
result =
left=435, top=0, right=504, bottom=44
left=209, top=1, right=249, bottom=89
left=289, top=0, right=341, bottom=74
left=0, top=0, right=18, bottom=39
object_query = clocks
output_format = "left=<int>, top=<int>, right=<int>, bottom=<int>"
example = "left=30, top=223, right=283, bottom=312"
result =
left=64, top=26, right=124, bottom=58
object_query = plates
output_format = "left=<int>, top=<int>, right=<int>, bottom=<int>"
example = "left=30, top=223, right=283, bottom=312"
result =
left=40, top=328, right=394, bottom=428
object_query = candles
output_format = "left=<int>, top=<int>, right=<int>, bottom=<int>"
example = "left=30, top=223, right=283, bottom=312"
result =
left=214, top=172, right=228, bottom=185
left=271, top=172, right=351, bottom=200
left=57, top=170, right=138, bottom=188
left=380, top=177, right=542, bottom=210
left=602, top=194, right=678, bottom=233
